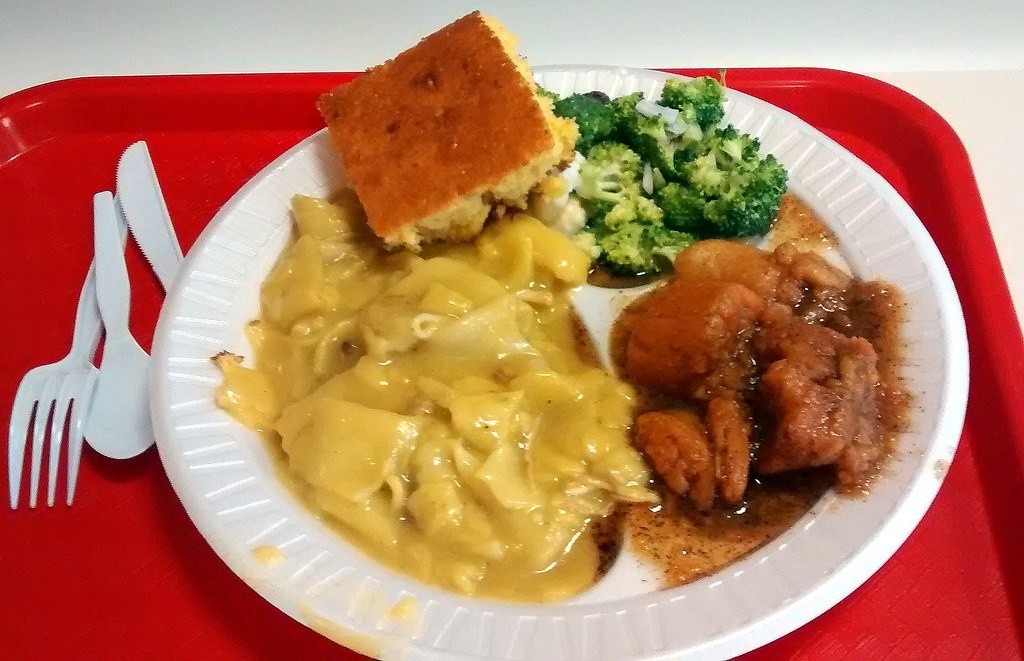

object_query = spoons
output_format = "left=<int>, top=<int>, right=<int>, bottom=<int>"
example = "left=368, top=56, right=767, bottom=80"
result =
left=84, top=190, right=154, bottom=460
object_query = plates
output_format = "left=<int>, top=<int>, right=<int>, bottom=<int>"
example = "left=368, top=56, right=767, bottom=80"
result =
left=151, top=62, right=970, bottom=661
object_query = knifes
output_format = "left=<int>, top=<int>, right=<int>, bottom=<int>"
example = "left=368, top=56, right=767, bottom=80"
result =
left=115, top=141, right=184, bottom=294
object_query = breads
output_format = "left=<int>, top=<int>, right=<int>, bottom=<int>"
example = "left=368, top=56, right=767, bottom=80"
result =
left=315, top=9, right=592, bottom=244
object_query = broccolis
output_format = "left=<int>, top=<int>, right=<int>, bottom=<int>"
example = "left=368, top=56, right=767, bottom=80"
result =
left=548, top=75, right=790, bottom=277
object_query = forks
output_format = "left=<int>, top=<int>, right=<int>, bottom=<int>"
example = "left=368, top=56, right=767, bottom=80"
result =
left=8, top=190, right=129, bottom=509
